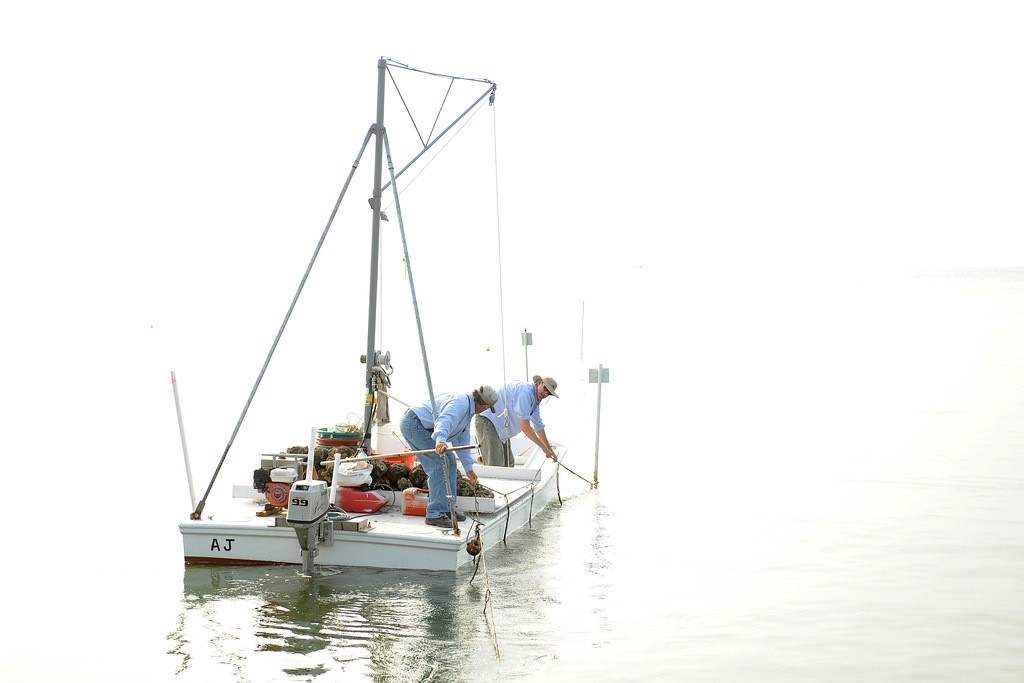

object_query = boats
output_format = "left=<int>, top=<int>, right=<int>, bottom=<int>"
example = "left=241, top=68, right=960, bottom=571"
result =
left=169, top=56, right=610, bottom=580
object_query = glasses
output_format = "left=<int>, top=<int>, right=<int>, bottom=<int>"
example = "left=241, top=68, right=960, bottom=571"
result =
left=542, top=382, right=552, bottom=395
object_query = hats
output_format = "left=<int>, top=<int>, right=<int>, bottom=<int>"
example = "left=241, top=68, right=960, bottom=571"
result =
left=532, top=375, right=559, bottom=398
left=476, top=385, right=499, bottom=413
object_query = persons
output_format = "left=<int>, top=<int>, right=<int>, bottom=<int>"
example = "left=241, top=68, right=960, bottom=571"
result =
left=400, top=385, right=497, bottom=526
left=474, top=375, right=559, bottom=468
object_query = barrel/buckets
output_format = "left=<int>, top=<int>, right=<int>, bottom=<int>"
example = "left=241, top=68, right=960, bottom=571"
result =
left=316, top=425, right=362, bottom=452
left=373, top=422, right=417, bottom=471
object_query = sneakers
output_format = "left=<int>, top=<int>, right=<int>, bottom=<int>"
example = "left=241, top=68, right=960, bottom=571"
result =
left=425, top=510, right=466, bottom=528
